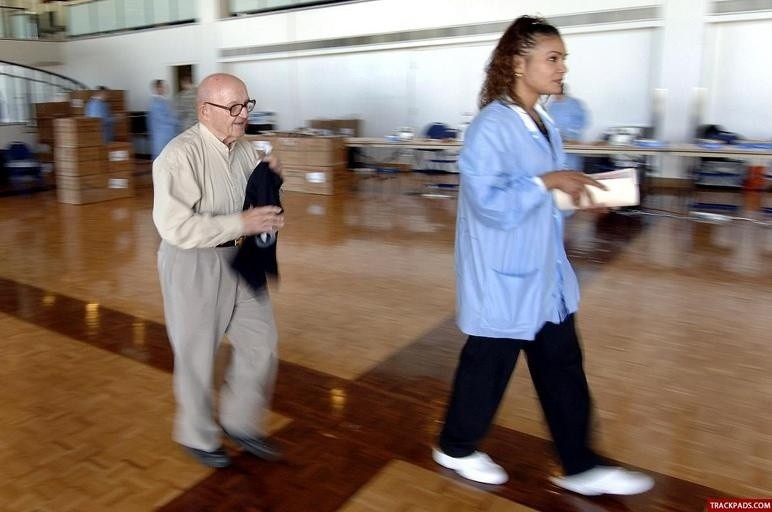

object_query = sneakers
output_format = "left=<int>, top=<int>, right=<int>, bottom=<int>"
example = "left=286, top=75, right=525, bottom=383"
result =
left=191, top=443, right=231, bottom=467
left=218, top=418, right=283, bottom=462
left=432, top=444, right=509, bottom=484
left=565, top=466, right=655, bottom=497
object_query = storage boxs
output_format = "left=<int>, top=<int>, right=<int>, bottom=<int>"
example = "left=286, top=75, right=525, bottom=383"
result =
left=28, top=85, right=150, bottom=208
left=248, top=116, right=359, bottom=198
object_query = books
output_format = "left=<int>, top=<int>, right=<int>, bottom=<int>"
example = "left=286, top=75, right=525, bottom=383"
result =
left=553, top=167, right=643, bottom=210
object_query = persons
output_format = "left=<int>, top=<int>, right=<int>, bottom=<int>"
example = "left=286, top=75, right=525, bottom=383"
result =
left=151, top=72, right=288, bottom=468
left=148, top=80, right=188, bottom=158
left=84, top=84, right=127, bottom=143
left=168, top=76, right=199, bottom=129
left=428, top=12, right=656, bottom=496
left=548, top=82, right=590, bottom=174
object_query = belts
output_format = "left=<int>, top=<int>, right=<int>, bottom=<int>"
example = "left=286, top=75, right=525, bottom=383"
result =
left=216, top=238, right=243, bottom=248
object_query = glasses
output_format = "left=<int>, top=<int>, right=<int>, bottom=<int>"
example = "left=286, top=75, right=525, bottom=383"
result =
left=203, top=99, right=256, bottom=117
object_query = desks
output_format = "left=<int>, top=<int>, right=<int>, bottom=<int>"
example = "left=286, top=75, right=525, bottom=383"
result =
left=349, top=133, right=772, bottom=213
